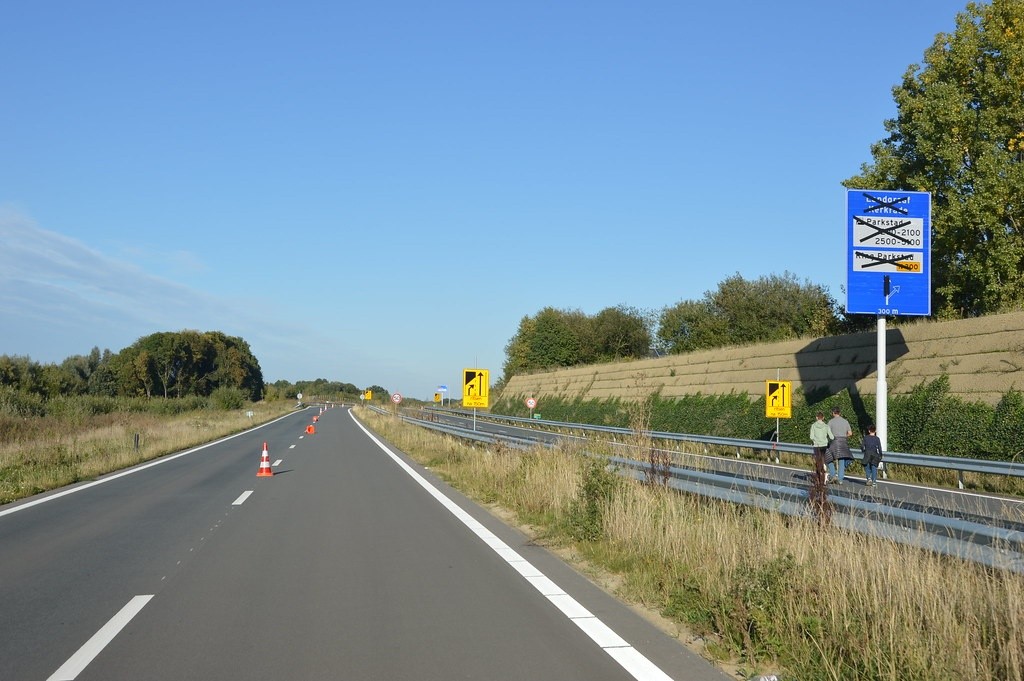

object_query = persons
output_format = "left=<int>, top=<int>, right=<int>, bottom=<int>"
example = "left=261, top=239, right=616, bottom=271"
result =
left=810, top=412, right=834, bottom=485
left=861, top=425, right=883, bottom=487
left=824, top=407, right=855, bottom=485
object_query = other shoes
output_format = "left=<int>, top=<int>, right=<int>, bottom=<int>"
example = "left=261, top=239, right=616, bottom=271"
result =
left=872, top=483, right=876, bottom=487
left=836, top=479, right=843, bottom=485
left=866, top=477, right=872, bottom=486
left=826, top=475, right=836, bottom=483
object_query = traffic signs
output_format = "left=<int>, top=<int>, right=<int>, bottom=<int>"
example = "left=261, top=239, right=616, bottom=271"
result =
left=847, top=189, right=932, bottom=315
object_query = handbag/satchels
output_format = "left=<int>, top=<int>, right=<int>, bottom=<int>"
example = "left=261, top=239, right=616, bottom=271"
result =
left=877, top=461, right=884, bottom=470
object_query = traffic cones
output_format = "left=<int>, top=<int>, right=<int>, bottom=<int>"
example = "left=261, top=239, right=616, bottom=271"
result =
left=306, top=424, right=315, bottom=434
left=256, top=439, right=274, bottom=477
